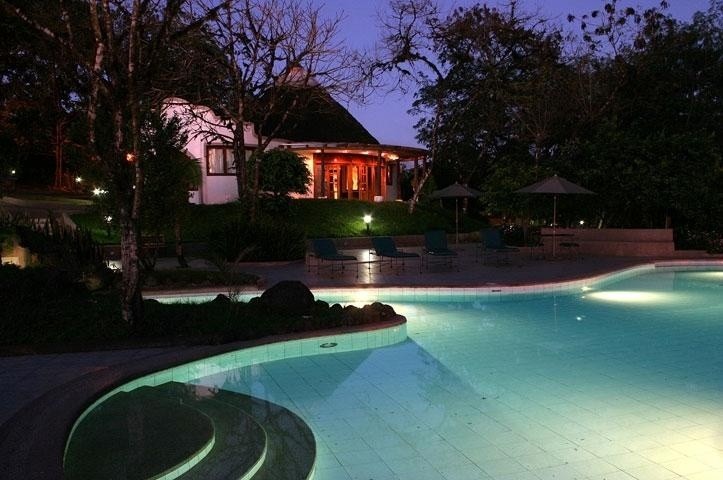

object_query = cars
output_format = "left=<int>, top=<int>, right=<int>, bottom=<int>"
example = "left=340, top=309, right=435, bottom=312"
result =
left=491, top=219, right=544, bottom=246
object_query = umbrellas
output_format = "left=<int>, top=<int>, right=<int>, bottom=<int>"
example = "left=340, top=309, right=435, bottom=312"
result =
left=511, top=173, right=598, bottom=255
left=425, top=180, right=503, bottom=246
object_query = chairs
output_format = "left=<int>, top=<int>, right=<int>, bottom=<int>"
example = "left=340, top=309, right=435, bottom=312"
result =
left=309, top=226, right=583, bottom=282
